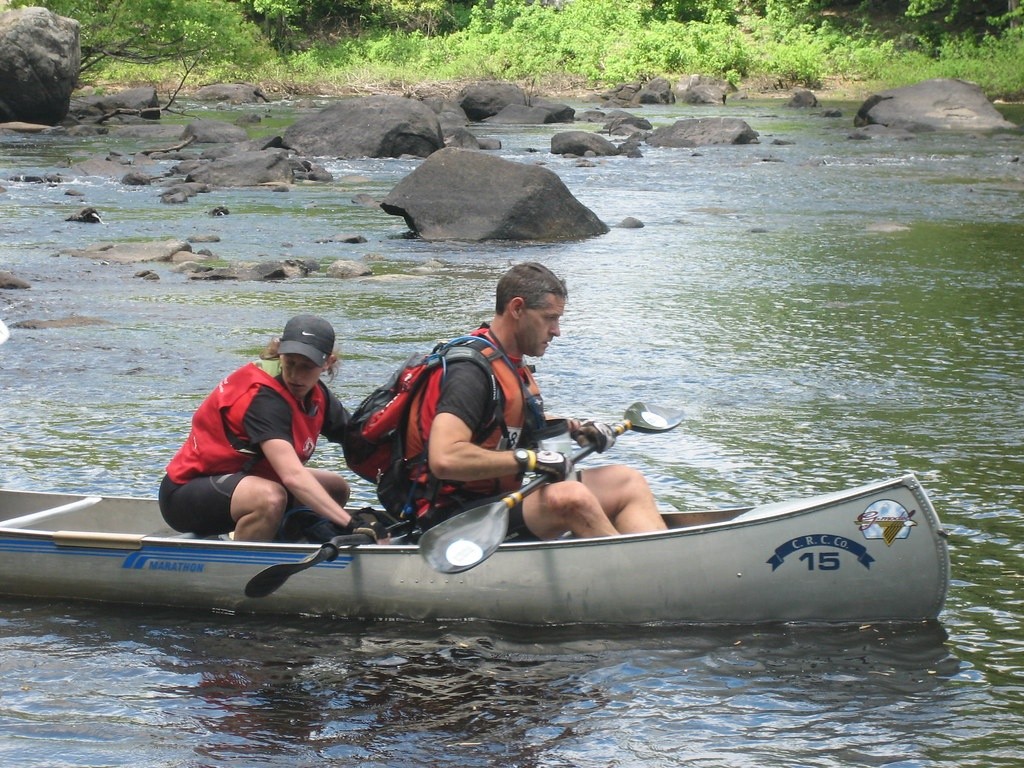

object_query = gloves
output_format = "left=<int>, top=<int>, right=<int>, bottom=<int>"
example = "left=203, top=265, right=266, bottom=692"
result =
left=525, top=448, right=574, bottom=483
left=569, top=417, right=616, bottom=454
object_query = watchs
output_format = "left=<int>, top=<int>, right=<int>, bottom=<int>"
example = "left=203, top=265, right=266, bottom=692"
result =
left=513, top=448, right=528, bottom=478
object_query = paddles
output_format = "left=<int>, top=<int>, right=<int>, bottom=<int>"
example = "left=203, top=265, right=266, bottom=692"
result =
left=417, top=399, right=685, bottom=576
left=242, top=520, right=412, bottom=602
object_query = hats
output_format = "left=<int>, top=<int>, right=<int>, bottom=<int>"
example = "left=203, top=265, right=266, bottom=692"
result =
left=278, top=314, right=335, bottom=367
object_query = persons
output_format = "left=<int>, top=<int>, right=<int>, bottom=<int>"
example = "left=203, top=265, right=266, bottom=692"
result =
left=159, top=315, right=390, bottom=545
left=408, top=262, right=668, bottom=543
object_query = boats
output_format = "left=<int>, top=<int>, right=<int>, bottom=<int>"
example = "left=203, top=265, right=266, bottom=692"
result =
left=0, top=473, right=954, bottom=627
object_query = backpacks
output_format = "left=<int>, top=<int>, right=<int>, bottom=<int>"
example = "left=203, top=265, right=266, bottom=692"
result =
left=343, top=347, right=500, bottom=485
